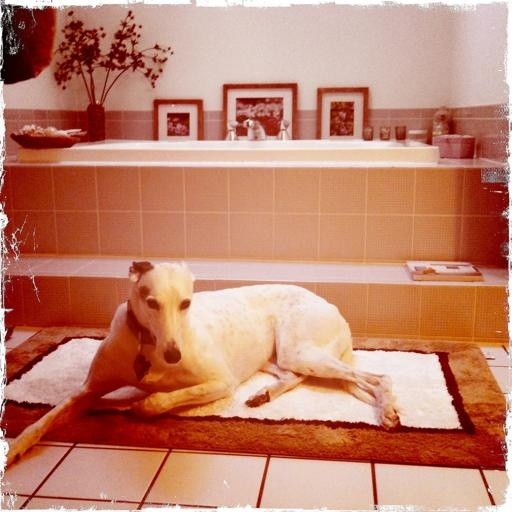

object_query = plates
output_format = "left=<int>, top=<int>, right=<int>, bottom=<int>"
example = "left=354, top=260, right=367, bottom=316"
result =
left=11, top=135, right=83, bottom=149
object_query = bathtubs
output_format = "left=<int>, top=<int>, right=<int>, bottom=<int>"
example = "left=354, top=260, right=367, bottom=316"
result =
left=60, top=138, right=441, bottom=164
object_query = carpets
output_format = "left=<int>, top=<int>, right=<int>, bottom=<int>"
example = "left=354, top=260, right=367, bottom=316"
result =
left=8, top=327, right=508, bottom=470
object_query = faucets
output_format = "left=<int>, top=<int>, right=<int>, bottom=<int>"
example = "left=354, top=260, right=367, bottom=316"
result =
left=243, top=120, right=266, bottom=141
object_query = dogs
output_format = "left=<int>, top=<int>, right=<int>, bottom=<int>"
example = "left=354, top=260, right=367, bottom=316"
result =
left=5, top=259, right=402, bottom=470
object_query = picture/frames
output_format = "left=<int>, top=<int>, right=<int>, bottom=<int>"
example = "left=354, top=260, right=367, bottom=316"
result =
left=221, top=82, right=297, bottom=141
left=316, top=86, right=369, bottom=139
left=152, top=97, right=204, bottom=141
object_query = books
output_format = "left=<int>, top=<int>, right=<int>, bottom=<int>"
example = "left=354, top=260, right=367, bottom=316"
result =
left=406, top=259, right=484, bottom=282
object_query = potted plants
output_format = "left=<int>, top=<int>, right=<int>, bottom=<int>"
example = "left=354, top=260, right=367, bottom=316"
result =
left=51, top=11, right=174, bottom=140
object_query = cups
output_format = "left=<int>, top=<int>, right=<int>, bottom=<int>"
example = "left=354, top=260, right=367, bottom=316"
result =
left=363, top=125, right=406, bottom=141
